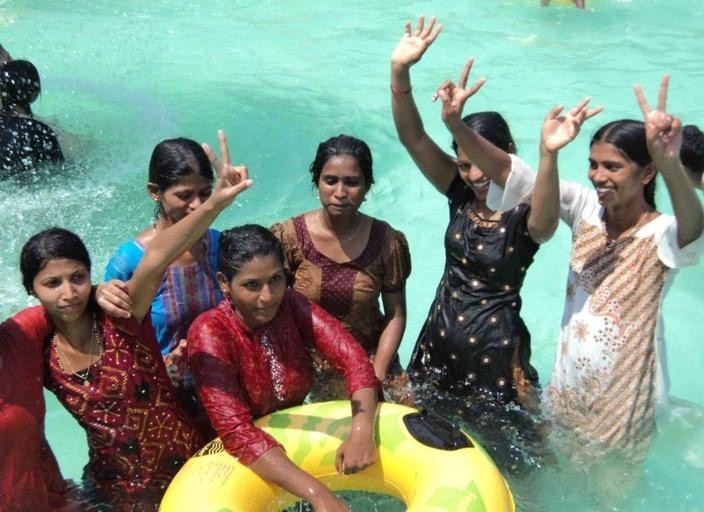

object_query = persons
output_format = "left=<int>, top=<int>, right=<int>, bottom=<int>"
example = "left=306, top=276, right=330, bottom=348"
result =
left=20, top=129, right=252, bottom=512
left=388, top=15, right=557, bottom=482
left=187, top=224, right=381, bottom=511
left=0, top=276, right=132, bottom=512
left=104, top=136, right=224, bottom=442
left=431, top=55, right=703, bottom=512
left=269, top=134, right=409, bottom=402
left=1, top=60, right=65, bottom=182
left=678, top=125, right=702, bottom=191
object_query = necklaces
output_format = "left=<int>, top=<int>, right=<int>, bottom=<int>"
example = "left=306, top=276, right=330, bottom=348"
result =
left=316, top=212, right=364, bottom=245
left=56, top=333, right=94, bottom=379
left=52, top=311, right=102, bottom=372
left=629, top=207, right=650, bottom=235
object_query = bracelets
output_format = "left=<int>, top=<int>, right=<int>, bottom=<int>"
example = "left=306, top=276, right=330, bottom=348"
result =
left=391, top=87, right=413, bottom=96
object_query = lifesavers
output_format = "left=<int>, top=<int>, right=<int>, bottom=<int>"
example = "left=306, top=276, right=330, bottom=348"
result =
left=157, top=399, right=516, bottom=511
